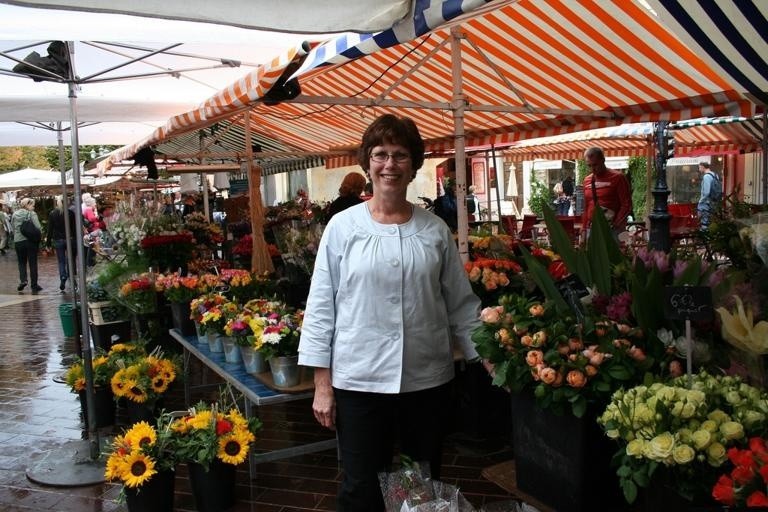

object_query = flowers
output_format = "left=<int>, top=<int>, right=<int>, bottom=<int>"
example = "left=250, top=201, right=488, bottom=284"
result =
left=388, top=453, right=433, bottom=512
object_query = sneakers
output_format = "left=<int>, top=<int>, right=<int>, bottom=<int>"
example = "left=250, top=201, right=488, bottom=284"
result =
left=17, top=281, right=27, bottom=291
left=60, top=276, right=67, bottom=290
left=31, top=285, right=43, bottom=290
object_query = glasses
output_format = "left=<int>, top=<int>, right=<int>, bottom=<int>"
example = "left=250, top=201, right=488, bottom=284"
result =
left=370, top=153, right=411, bottom=162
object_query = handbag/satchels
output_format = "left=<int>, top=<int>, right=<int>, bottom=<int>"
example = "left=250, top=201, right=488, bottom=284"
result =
left=20, top=220, right=41, bottom=245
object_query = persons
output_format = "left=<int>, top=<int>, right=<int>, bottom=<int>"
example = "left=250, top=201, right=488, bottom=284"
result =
left=329, top=172, right=374, bottom=217
left=159, top=193, right=195, bottom=221
left=580, top=151, right=632, bottom=250
left=697, top=161, right=719, bottom=231
left=432, top=179, right=457, bottom=232
left=552, top=183, right=576, bottom=215
left=47, top=193, right=107, bottom=290
left=1, top=198, right=41, bottom=292
left=297, top=114, right=511, bottom=512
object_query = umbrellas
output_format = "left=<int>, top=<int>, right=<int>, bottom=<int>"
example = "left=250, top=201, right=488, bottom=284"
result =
left=0, top=0, right=412, bottom=489
left=0, top=149, right=181, bottom=200
left=0, top=122, right=167, bottom=385
